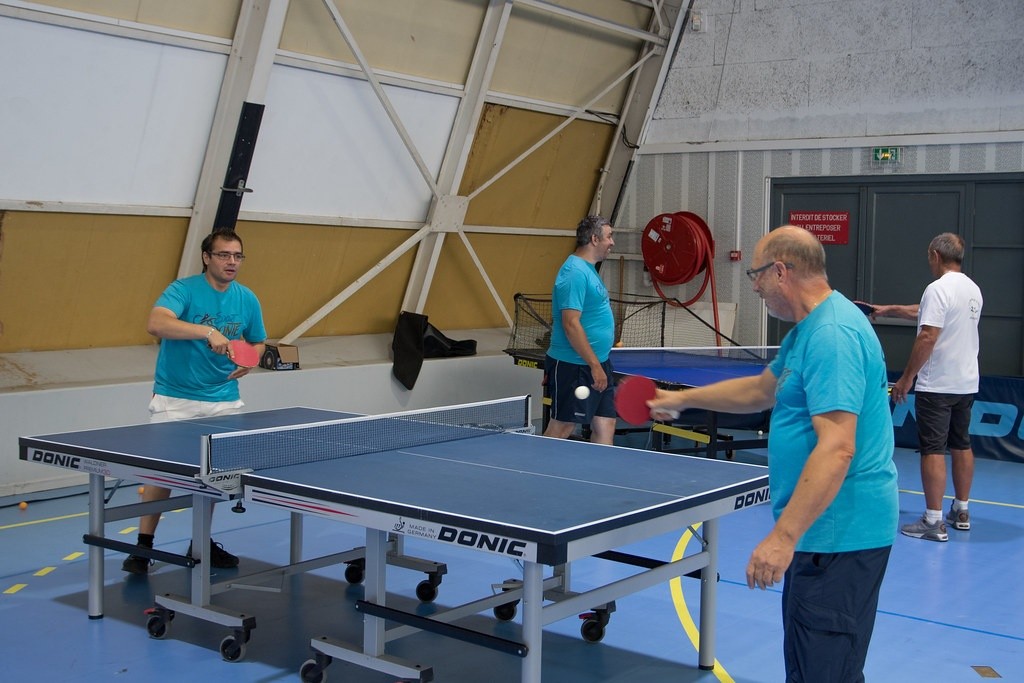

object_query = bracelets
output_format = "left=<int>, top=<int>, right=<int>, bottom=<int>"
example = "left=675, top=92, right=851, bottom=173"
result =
left=208, top=327, right=215, bottom=340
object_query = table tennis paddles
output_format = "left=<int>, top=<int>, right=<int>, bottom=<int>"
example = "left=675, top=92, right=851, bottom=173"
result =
left=615, top=376, right=681, bottom=425
left=852, top=300, right=876, bottom=316
left=206, top=339, right=259, bottom=367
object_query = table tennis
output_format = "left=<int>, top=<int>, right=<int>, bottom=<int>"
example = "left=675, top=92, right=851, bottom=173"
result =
left=574, top=386, right=590, bottom=400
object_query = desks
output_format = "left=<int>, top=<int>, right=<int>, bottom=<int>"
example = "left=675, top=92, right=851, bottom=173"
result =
left=503, top=348, right=897, bottom=466
left=17, top=407, right=770, bottom=683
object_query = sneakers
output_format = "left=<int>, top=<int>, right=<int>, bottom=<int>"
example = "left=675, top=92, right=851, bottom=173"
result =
left=900, top=516, right=948, bottom=541
left=186, top=538, right=237, bottom=568
left=121, top=541, right=153, bottom=572
left=945, top=500, right=970, bottom=530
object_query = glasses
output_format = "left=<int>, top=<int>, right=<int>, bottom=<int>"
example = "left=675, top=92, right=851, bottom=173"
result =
left=211, top=251, right=245, bottom=262
left=746, top=262, right=793, bottom=281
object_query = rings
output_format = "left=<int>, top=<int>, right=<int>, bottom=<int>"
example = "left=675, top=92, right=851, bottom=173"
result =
left=897, top=395, right=902, bottom=397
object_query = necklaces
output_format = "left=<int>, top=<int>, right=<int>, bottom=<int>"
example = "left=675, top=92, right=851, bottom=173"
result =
left=813, top=290, right=831, bottom=310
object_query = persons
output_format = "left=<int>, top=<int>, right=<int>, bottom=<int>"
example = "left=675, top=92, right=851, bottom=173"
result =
left=544, top=214, right=617, bottom=446
left=647, top=224, right=899, bottom=683
left=872, top=233, right=982, bottom=543
left=116, top=229, right=265, bottom=571
